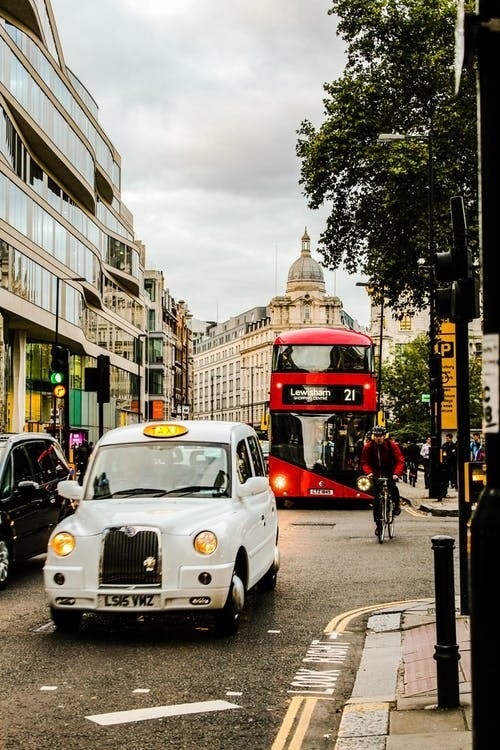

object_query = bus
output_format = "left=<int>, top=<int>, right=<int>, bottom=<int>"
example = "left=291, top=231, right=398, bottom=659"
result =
left=260, top=327, right=389, bottom=506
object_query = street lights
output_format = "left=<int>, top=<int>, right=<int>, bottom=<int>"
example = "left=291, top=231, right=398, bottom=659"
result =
left=54, top=276, right=88, bottom=344
left=135, top=333, right=149, bottom=423
left=354, top=282, right=385, bottom=424
left=240, top=366, right=264, bottom=428
left=210, top=375, right=221, bottom=419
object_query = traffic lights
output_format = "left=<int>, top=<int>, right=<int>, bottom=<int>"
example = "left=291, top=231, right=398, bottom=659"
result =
left=51, top=345, right=69, bottom=401
left=435, top=250, right=461, bottom=320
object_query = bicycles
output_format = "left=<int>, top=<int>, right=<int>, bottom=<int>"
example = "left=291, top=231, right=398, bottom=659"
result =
left=368, top=477, right=402, bottom=544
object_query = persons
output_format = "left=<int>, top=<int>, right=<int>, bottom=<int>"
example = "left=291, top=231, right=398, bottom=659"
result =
left=361, top=425, right=404, bottom=536
left=271, top=416, right=364, bottom=477
left=383, top=432, right=485, bottom=527
left=77, top=440, right=95, bottom=486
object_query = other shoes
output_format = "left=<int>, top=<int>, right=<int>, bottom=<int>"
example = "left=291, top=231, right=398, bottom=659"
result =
left=393, top=506, right=402, bottom=516
left=375, top=526, right=382, bottom=536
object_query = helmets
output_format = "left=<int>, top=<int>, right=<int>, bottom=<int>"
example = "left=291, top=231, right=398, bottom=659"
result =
left=372, top=425, right=386, bottom=435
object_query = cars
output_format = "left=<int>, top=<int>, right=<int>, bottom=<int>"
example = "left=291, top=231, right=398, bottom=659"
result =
left=0, top=432, right=82, bottom=588
left=43, top=419, right=282, bottom=640
left=260, top=440, right=269, bottom=464
